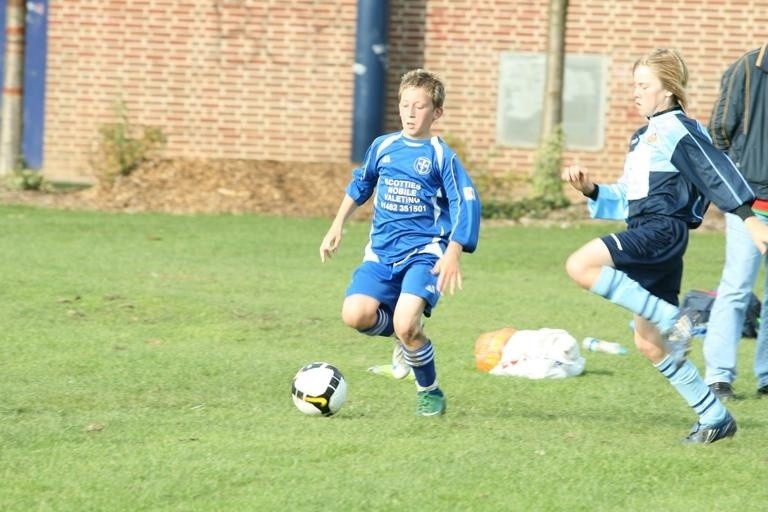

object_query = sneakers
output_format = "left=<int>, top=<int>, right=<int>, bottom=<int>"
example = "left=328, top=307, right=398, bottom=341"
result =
left=391, top=340, right=410, bottom=381
left=663, top=309, right=702, bottom=364
left=709, top=382, right=735, bottom=400
left=419, top=393, right=448, bottom=419
left=681, top=410, right=737, bottom=443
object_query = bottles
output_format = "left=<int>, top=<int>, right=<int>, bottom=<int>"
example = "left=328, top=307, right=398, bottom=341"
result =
left=583, top=336, right=630, bottom=359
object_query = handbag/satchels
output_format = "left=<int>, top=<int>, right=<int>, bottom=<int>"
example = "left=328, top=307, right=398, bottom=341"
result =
left=488, top=327, right=586, bottom=379
left=679, top=290, right=762, bottom=338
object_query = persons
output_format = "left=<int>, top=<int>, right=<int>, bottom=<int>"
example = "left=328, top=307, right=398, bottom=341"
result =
left=318, top=68, right=482, bottom=418
left=559, top=45, right=767, bottom=444
left=703, top=45, right=768, bottom=396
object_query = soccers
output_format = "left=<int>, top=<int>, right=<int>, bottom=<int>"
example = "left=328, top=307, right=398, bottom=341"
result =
left=292, top=362, right=345, bottom=416
